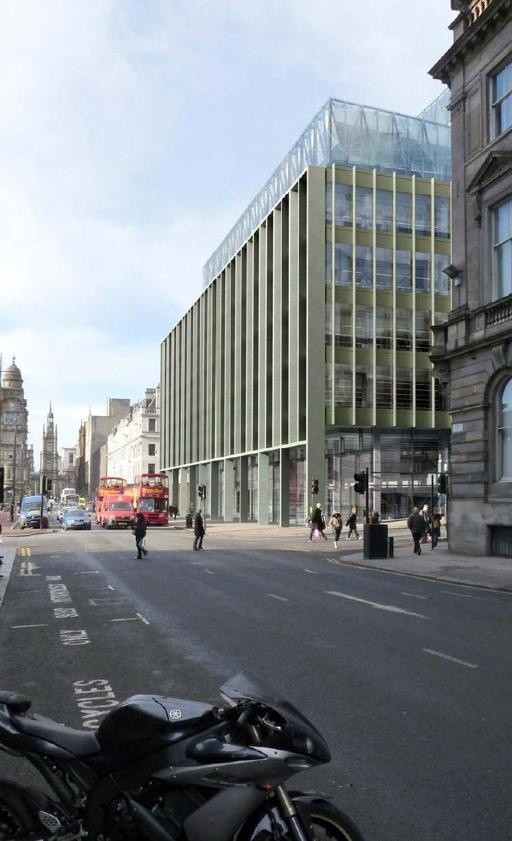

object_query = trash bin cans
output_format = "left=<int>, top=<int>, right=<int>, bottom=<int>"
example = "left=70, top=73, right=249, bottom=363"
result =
left=363, top=524, right=388, bottom=560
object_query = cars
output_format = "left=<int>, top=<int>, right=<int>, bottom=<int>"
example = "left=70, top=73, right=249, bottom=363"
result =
left=47, top=494, right=94, bottom=521
left=57, top=502, right=91, bottom=529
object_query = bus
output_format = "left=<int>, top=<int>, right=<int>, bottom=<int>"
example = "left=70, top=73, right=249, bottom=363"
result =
left=94, top=476, right=127, bottom=525
left=60, top=487, right=76, bottom=505
left=125, top=472, right=170, bottom=526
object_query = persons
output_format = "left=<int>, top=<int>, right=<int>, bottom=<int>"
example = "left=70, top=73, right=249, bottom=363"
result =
left=406, top=503, right=442, bottom=556
left=346, top=507, right=362, bottom=542
left=131, top=512, right=150, bottom=562
left=193, top=509, right=206, bottom=552
left=174, top=504, right=178, bottom=520
left=307, top=503, right=326, bottom=544
left=327, top=511, right=343, bottom=551
left=169, top=503, right=174, bottom=519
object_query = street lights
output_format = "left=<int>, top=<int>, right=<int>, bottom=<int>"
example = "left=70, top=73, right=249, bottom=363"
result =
left=8, top=411, right=20, bottom=519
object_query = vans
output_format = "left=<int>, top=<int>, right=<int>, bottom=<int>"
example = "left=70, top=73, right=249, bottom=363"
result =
left=20, top=496, right=49, bottom=529
left=101, top=493, right=135, bottom=529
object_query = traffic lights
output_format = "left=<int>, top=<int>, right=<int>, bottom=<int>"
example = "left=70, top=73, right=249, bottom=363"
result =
left=198, top=485, right=204, bottom=497
left=354, top=469, right=367, bottom=493
left=46, top=478, right=53, bottom=490
left=437, top=473, right=446, bottom=494
left=311, top=478, right=320, bottom=493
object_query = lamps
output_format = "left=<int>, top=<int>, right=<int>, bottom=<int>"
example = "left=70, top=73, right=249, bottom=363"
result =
left=443, top=263, right=463, bottom=288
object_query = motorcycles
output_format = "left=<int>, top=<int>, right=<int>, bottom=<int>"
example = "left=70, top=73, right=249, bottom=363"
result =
left=0, top=670, right=367, bottom=840
